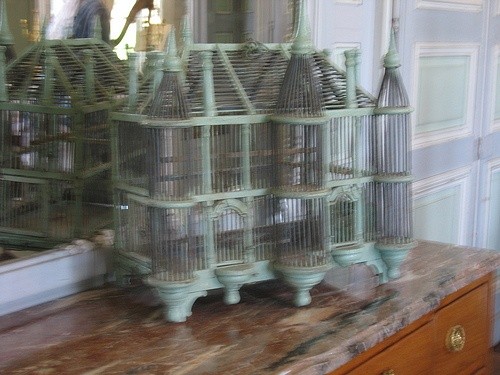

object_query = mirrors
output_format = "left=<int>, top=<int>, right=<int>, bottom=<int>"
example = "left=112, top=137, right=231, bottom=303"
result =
left=0, top=0, right=303, bottom=270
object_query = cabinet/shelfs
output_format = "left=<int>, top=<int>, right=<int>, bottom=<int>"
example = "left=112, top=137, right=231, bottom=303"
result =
left=0, top=220, right=500, bottom=374
